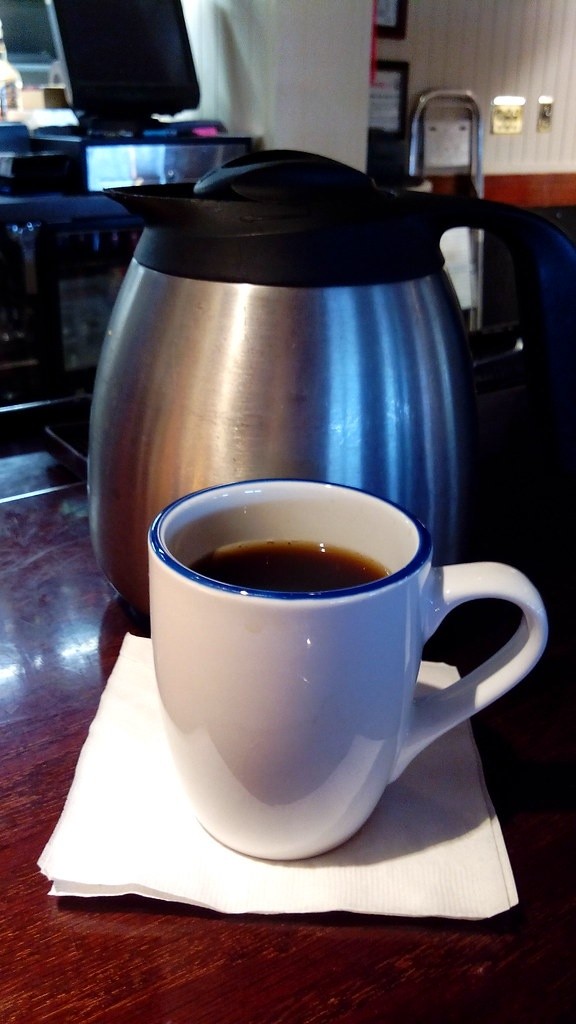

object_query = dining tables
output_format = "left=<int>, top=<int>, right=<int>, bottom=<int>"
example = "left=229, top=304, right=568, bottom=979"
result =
left=0, top=474, right=576, bottom=1023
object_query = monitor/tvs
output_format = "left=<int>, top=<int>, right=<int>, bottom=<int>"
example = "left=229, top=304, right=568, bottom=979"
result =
left=46, top=0, right=201, bottom=138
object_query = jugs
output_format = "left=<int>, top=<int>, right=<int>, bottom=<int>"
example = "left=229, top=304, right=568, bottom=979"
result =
left=85, top=148, right=575, bottom=620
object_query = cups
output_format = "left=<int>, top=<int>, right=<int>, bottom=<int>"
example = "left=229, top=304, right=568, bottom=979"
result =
left=148, top=476, right=550, bottom=862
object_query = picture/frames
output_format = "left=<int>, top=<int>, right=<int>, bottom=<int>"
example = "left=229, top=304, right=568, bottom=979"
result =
left=373, top=0, right=408, bottom=41
left=369, top=60, right=409, bottom=141
